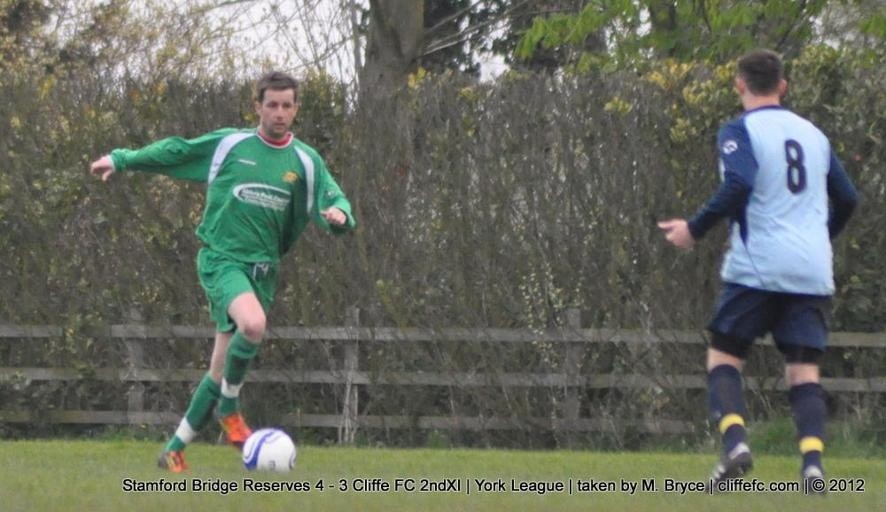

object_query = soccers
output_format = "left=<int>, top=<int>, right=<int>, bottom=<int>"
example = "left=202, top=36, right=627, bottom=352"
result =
left=242, top=429, right=296, bottom=472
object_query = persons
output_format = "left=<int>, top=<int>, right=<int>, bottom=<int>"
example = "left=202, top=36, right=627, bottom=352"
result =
left=656, top=46, right=861, bottom=501
left=84, top=68, right=361, bottom=475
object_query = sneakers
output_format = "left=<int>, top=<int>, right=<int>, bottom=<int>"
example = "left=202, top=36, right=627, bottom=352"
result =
left=705, top=442, right=753, bottom=492
left=213, top=406, right=254, bottom=451
left=802, top=465, right=828, bottom=496
left=157, top=449, right=192, bottom=477
